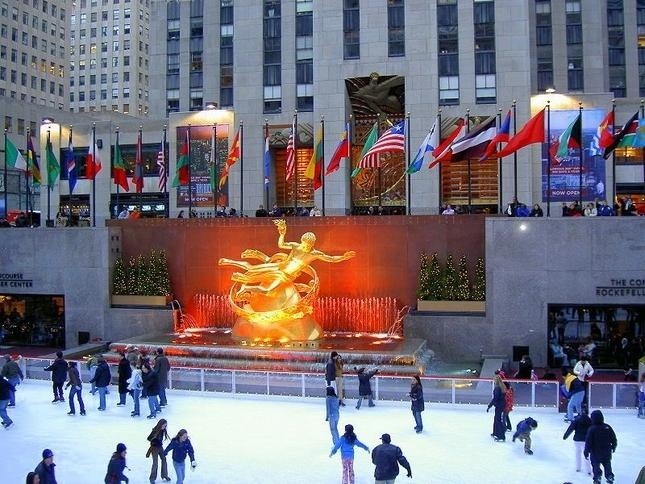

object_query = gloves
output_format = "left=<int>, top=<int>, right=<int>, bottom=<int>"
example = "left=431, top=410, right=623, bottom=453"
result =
left=191, top=461, right=196, bottom=467
left=76, top=386, right=80, bottom=390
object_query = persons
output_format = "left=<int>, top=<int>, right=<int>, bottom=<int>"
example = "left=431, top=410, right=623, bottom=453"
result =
left=0, top=304, right=198, bottom=484
left=217, top=220, right=357, bottom=297
left=355, top=71, right=401, bottom=112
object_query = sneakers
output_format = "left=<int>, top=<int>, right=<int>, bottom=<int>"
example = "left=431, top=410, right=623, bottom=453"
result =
left=7, top=403, right=14, bottom=405
left=98, top=407, right=105, bottom=409
left=52, top=396, right=65, bottom=402
left=117, top=402, right=125, bottom=405
left=356, top=404, right=375, bottom=409
left=339, top=400, right=346, bottom=406
left=131, top=394, right=167, bottom=418
left=525, top=448, right=533, bottom=454
left=1, top=421, right=12, bottom=428
left=563, top=412, right=581, bottom=422
left=491, top=426, right=511, bottom=440
left=151, top=474, right=170, bottom=484
left=414, top=426, right=422, bottom=432
left=68, top=410, right=85, bottom=414
left=593, top=478, right=614, bottom=484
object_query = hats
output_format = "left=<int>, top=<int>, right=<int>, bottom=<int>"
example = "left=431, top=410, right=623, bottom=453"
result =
left=379, top=434, right=390, bottom=440
left=117, top=444, right=126, bottom=453
left=331, top=351, right=337, bottom=357
left=43, top=449, right=53, bottom=458
